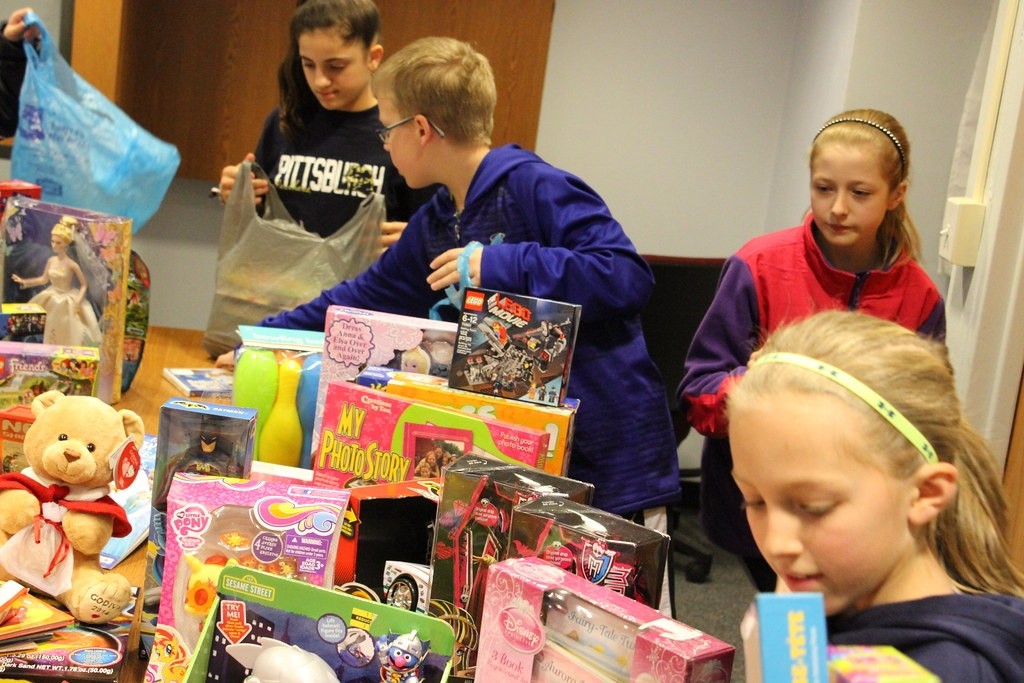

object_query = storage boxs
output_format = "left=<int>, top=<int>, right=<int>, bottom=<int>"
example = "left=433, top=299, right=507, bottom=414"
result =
left=0, top=180, right=736, bottom=683
left=740, top=593, right=828, bottom=683
left=827, top=644, right=942, bottom=683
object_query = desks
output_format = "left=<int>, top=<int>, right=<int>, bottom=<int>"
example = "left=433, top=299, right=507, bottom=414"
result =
left=0, top=326, right=240, bottom=683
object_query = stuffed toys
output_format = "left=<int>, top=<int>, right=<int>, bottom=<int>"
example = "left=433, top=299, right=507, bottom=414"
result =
left=0, top=390, right=144, bottom=623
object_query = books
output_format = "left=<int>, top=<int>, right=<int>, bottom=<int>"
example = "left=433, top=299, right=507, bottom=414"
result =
left=162, top=367, right=234, bottom=397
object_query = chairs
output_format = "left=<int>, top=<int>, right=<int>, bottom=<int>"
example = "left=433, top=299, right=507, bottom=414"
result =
left=639, top=255, right=726, bottom=583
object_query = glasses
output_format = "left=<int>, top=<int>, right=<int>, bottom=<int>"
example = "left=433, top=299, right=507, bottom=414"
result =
left=376, top=114, right=446, bottom=143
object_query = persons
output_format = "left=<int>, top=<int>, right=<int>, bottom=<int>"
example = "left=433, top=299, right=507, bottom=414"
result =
left=0, top=7, right=42, bottom=140
left=674, top=110, right=946, bottom=592
left=218, top=0, right=440, bottom=259
left=9, top=215, right=101, bottom=346
left=177, top=415, right=244, bottom=478
left=415, top=444, right=459, bottom=478
left=722, top=309, right=1023, bottom=683
left=213, top=35, right=678, bottom=619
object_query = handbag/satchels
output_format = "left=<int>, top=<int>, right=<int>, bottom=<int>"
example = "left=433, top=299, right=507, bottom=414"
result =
left=9, top=10, right=183, bottom=239
left=202, top=159, right=387, bottom=361
